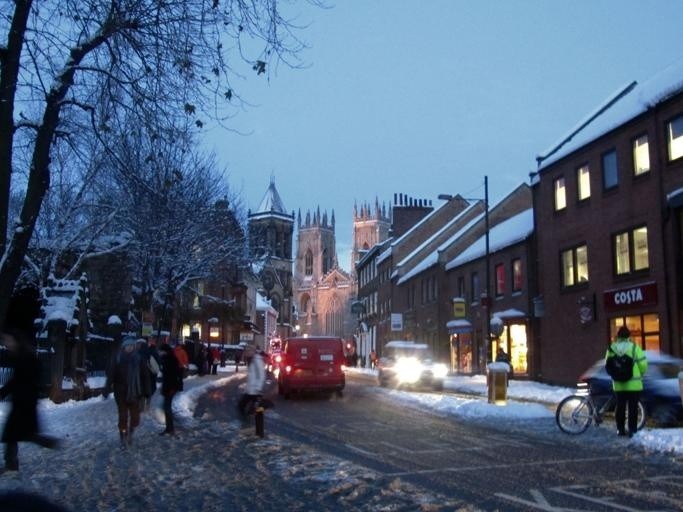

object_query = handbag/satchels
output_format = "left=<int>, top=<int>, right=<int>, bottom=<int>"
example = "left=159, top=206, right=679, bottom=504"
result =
left=147, top=354, right=159, bottom=374
left=245, top=394, right=274, bottom=416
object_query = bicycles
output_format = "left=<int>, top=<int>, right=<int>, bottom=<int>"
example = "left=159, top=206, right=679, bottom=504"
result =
left=555, top=376, right=647, bottom=435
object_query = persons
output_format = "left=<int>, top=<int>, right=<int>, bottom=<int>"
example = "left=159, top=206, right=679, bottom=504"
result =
left=0, top=325, right=64, bottom=476
left=173, top=343, right=189, bottom=392
left=237, top=342, right=273, bottom=434
left=352, top=351, right=358, bottom=367
left=101, top=336, right=152, bottom=453
left=211, top=347, right=220, bottom=375
left=368, top=350, right=377, bottom=369
left=157, top=344, right=184, bottom=437
left=605, top=325, right=647, bottom=439
left=135, top=336, right=159, bottom=425
left=496, top=348, right=508, bottom=387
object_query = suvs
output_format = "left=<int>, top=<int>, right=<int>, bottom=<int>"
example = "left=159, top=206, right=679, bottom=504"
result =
left=276, top=336, right=346, bottom=399
left=377, top=341, right=443, bottom=392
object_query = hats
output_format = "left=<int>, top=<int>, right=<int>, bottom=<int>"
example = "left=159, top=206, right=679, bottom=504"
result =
left=617, top=326, right=631, bottom=338
left=121, top=335, right=136, bottom=347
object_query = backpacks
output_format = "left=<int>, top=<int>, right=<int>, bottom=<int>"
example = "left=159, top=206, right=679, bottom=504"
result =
left=605, top=342, right=636, bottom=383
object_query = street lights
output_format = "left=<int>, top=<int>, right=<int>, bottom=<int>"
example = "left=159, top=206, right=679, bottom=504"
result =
left=436, top=175, right=494, bottom=362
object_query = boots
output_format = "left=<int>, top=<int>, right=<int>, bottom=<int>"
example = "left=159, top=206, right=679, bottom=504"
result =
left=129, top=425, right=136, bottom=442
left=119, top=429, right=128, bottom=450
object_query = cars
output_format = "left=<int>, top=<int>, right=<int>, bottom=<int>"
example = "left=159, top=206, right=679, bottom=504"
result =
left=580, top=350, right=683, bottom=428
